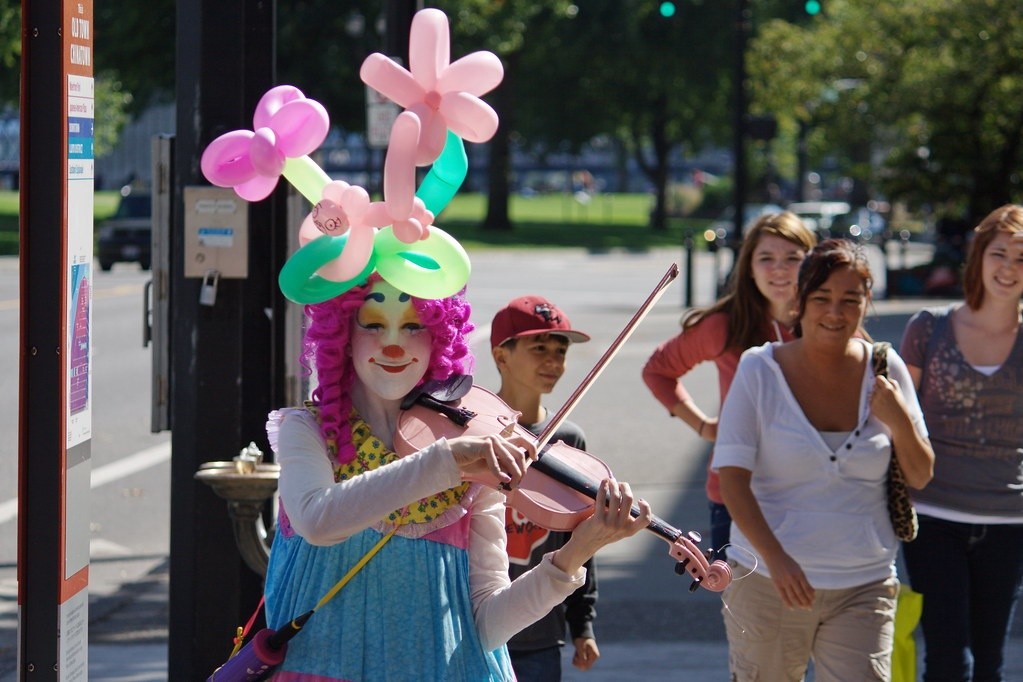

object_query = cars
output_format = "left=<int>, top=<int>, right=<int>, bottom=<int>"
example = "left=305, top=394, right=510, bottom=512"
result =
left=99, top=189, right=150, bottom=275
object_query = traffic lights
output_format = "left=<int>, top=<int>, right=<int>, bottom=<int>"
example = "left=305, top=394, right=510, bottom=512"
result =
left=655, top=0, right=698, bottom=24
left=781, top=0, right=824, bottom=25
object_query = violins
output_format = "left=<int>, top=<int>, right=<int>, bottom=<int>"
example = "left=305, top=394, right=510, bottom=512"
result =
left=394, top=374, right=759, bottom=593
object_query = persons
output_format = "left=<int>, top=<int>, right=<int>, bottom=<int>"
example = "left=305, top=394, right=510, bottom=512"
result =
left=643, top=213, right=816, bottom=561
left=256, top=272, right=650, bottom=682
left=488, top=295, right=601, bottom=682
left=900, top=203, right=1023, bottom=682
left=710, top=239, right=934, bottom=682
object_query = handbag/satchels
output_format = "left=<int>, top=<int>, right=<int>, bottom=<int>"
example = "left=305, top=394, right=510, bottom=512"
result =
left=872, top=338, right=921, bottom=542
left=891, top=583, right=925, bottom=682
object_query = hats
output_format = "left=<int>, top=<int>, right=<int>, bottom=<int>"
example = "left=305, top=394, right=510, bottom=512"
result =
left=490, top=296, right=590, bottom=350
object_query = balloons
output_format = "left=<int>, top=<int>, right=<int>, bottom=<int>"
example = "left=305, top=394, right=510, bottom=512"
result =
left=201, top=8, right=505, bottom=305
